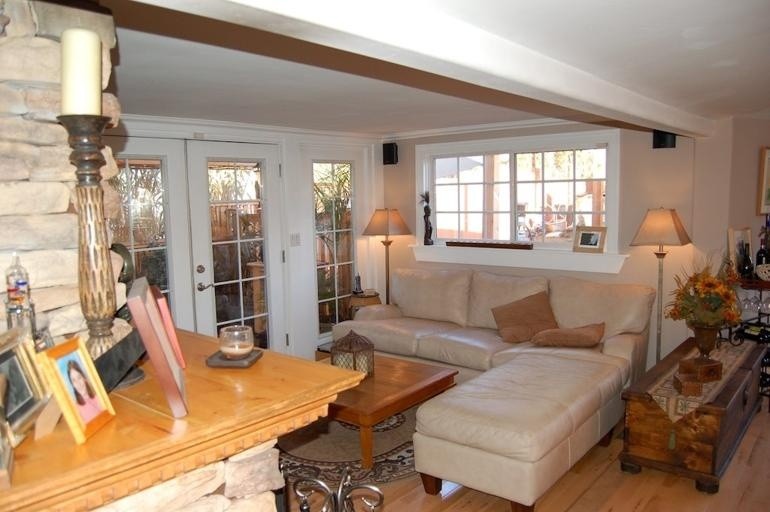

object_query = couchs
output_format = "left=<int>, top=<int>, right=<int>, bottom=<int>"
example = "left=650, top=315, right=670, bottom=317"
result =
left=333, top=261, right=656, bottom=512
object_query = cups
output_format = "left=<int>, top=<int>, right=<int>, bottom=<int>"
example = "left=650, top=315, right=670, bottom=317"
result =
left=217, top=325, right=255, bottom=361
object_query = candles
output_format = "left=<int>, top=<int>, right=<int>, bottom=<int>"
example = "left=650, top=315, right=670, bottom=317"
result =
left=60, top=28, right=101, bottom=115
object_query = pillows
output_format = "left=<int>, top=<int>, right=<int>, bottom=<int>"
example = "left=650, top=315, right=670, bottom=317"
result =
left=390, top=268, right=656, bottom=348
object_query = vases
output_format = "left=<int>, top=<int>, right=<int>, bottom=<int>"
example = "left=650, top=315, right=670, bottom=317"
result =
left=694, top=326, right=718, bottom=359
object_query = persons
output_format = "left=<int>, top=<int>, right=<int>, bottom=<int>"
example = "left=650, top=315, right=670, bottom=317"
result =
left=64, top=358, right=104, bottom=427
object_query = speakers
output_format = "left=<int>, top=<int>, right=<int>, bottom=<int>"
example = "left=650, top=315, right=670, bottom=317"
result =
left=652, top=129, right=676, bottom=148
left=383, top=143, right=398, bottom=164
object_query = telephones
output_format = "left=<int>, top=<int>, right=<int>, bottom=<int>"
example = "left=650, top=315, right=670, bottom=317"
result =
left=352, top=272, right=363, bottom=295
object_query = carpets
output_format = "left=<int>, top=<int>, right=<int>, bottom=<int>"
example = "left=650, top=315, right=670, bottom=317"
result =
left=274, top=402, right=434, bottom=492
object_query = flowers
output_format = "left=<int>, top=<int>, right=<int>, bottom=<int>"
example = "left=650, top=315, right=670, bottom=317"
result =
left=663, top=254, right=742, bottom=329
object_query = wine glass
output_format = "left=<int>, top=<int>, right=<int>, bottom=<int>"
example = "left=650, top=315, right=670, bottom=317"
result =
left=740, top=290, right=770, bottom=314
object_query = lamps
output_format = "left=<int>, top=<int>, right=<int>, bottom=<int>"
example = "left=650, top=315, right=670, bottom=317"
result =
left=629, top=208, right=692, bottom=364
left=362, top=209, right=412, bottom=305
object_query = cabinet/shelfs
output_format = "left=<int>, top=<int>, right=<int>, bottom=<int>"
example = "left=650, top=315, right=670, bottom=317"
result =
left=620, top=337, right=768, bottom=493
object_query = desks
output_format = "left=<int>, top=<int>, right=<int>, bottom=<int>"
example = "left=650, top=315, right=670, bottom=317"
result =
left=349, top=293, right=382, bottom=320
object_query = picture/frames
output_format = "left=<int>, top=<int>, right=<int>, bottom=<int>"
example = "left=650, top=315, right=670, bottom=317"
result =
left=0, top=276, right=188, bottom=446
left=728, top=226, right=753, bottom=280
left=755, top=144, right=770, bottom=216
left=573, top=226, right=607, bottom=253
